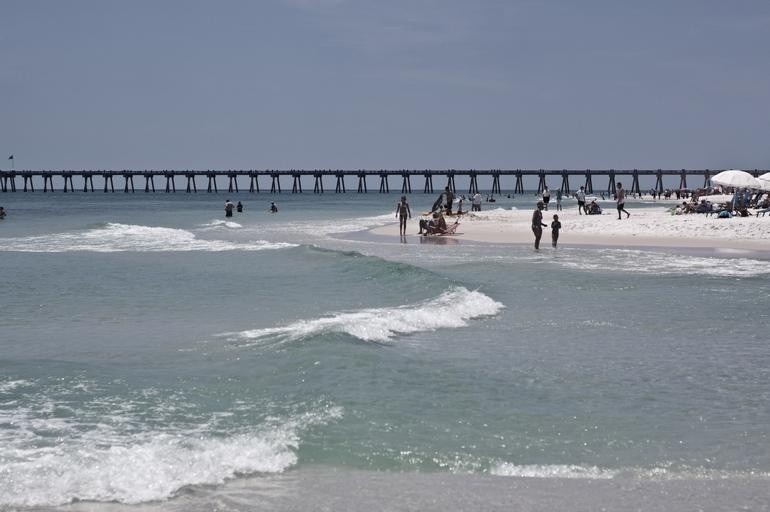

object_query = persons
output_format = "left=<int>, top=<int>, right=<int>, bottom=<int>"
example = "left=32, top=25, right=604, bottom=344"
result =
left=456, top=198, right=463, bottom=215
left=236, top=201, right=243, bottom=212
left=423, top=212, right=444, bottom=236
left=270, top=201, right=278, bottom=213
left=395, top=194, right=412, bottom=236
left=441, top=186, right=453, bottom=216
left=531, top=201, right=547, bottom=250
left=472, top=190, right=482, bottom=212
left=417, top=209, right=447, bottom=237
left=542, top=185, right=550, bottom=211
left=550, top=214, right=561, bottom=248
left=0, top=206, right=6, bottom=220
left=575, top=186, right=588, bottom=215
left=555, top=188, right=563, bottom=211
left=601, top=181, right=770, bottom=220
left=224, top=200, right=235, bottom=217
left=587, top=200, right=601, bottom=215
left=457, top=192, right=497, bottom=203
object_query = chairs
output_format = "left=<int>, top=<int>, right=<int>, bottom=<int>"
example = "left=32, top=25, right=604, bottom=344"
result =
left=430, top=221, right=460, bottom=236
left=706, top=201, right=770, bottom=218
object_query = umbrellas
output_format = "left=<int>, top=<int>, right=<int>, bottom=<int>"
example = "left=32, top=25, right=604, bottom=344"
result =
left=757, top=173, right=770, bottom=180
left=752, top=177, right=770, bottom=192
left=709, top=170, right=760, bottom=212
